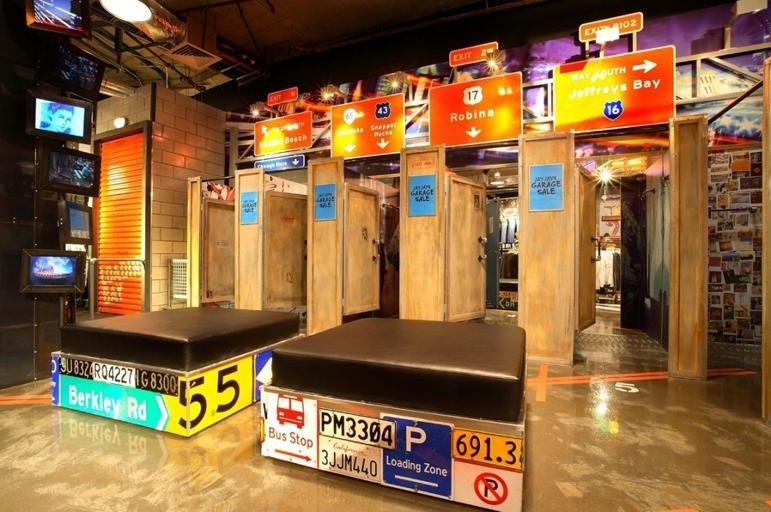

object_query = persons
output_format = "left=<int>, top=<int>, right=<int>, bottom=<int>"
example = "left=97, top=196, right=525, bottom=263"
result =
left=39, top=102, right=79, bottom=137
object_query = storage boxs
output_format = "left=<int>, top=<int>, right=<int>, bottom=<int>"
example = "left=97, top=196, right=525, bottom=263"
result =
left=48, top=298, right=299, bottom=441
left=258, top=311, right=528, bottom=512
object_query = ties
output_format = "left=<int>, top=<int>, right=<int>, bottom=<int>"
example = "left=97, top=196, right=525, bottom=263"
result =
left=40, top=36, right=106, bottom=100
left=45, top=144, right=101, bottom=197
left=19, top=249, right=86, bottom=294
left=24, top=89, right=92, bottom=145
left=58, top=201, right=93, bottom=245
left=25, top=0, right=91, bottom=38
